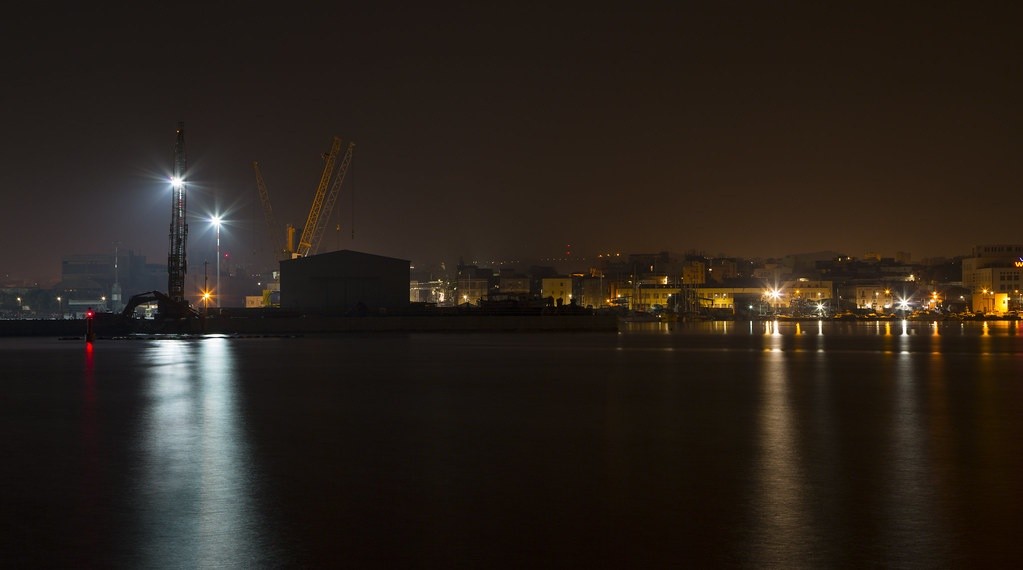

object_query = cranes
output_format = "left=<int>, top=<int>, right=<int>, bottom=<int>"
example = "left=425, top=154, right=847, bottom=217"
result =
left=252, top=134, right=357, bottom=309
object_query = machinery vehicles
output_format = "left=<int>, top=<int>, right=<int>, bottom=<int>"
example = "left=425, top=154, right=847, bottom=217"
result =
left=92, top=290, right=205, bottom=324
